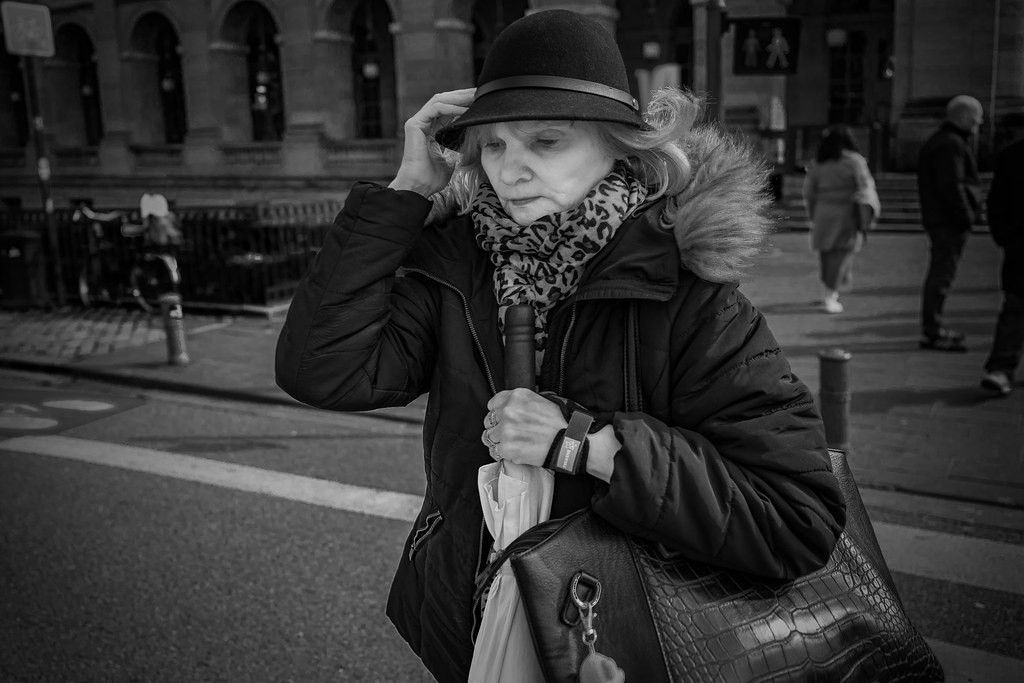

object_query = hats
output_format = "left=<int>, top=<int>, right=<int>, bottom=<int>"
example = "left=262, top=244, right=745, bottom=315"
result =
left=434, top=8, right=651, bottom=152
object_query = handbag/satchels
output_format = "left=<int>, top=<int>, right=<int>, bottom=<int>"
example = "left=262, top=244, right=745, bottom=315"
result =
left=471, top=290, right=945, bottom=683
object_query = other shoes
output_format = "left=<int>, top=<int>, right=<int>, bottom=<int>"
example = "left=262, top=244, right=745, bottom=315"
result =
left=939, top=328, right=965, bottom=341
left=824, top=296, right=842, bottom=313
left=919, top=339, right=968, bottom=354
left=981, top=371, right=1011, bottom=393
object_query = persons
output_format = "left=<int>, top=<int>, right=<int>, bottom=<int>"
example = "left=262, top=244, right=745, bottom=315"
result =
left=977, top=134, right=1024, bottom=400
left=918, top=94, right=985, bottom=353
left=277, top=8, right=850, bottom=682
left=801, top=124, right=883, bottom=315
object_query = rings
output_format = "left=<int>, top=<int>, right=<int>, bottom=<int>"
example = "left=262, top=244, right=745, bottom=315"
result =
left=488, top=409, right=498, bottom=426
left=484, top=428, right=497, bottom=446
left=489, top=444, right=504, bottom=461
